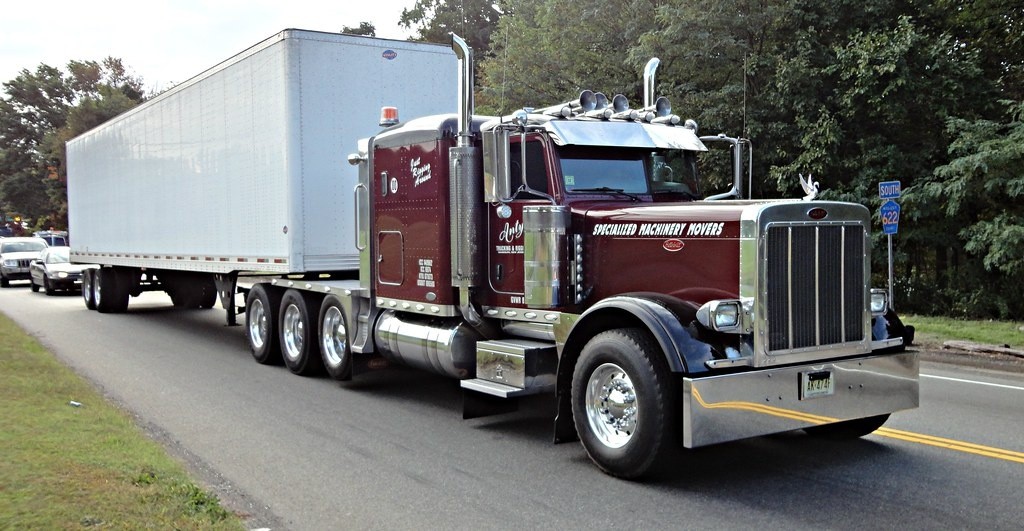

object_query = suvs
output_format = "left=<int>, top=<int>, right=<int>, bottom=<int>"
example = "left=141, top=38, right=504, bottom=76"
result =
left=0, top=236, right=51, bottom=288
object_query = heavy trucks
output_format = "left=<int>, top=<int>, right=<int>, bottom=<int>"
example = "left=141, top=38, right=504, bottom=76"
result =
left=66, top=28, right=923, bottom=478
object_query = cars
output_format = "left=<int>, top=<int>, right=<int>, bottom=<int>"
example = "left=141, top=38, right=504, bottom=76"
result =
left=29, top=246, right=100, bottom=295
